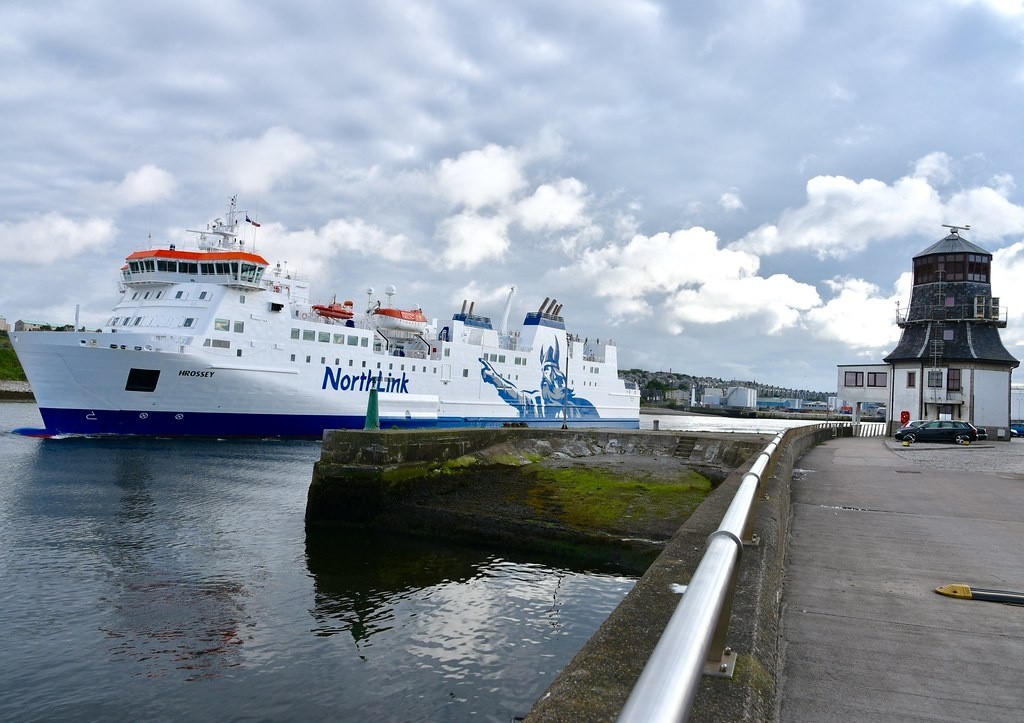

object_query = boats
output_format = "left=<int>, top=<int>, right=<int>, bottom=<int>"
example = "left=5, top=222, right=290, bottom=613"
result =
left=311, top=304, right=354, bottom=318
left=8, top=192, right=642, bottom=434
left=371, top=308, right=426, bottom=332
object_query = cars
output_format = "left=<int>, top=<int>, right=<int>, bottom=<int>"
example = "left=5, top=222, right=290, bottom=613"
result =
left=893, top=422, right=988, bottom=444
left=1010, top=425, right=1024, bottom=437
